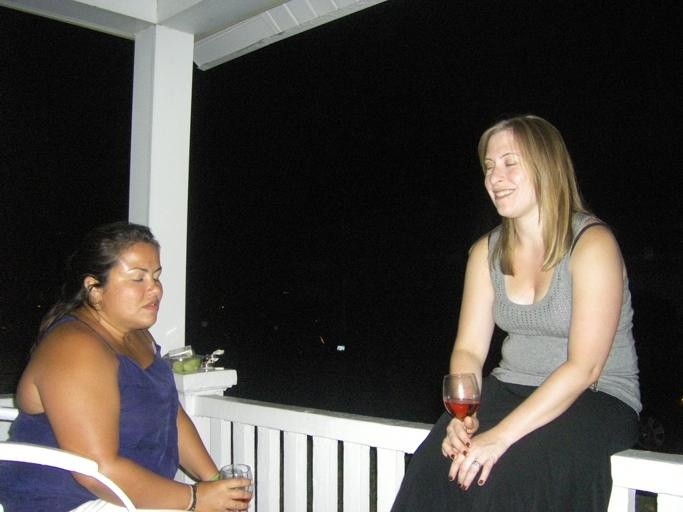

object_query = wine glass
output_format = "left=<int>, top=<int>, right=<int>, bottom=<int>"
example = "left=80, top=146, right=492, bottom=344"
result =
left=442, top=373, right=482, bottom=432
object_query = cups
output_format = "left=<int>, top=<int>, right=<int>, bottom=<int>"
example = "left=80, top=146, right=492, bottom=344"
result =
left=221, top=463, right=254, bottom=503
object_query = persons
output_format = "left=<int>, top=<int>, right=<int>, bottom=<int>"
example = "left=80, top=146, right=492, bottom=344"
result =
left=384, top=114, right=647, bottom=511
left=0, top=219, right=255, bottom=511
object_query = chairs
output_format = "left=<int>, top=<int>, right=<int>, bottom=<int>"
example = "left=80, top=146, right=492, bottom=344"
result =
left=0, top=406, right=138, bottom=512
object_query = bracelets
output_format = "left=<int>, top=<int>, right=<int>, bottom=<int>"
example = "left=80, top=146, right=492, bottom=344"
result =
left=206, top=471, right=221, bottom=481
left=185, top=483, right=197, bottom=511
